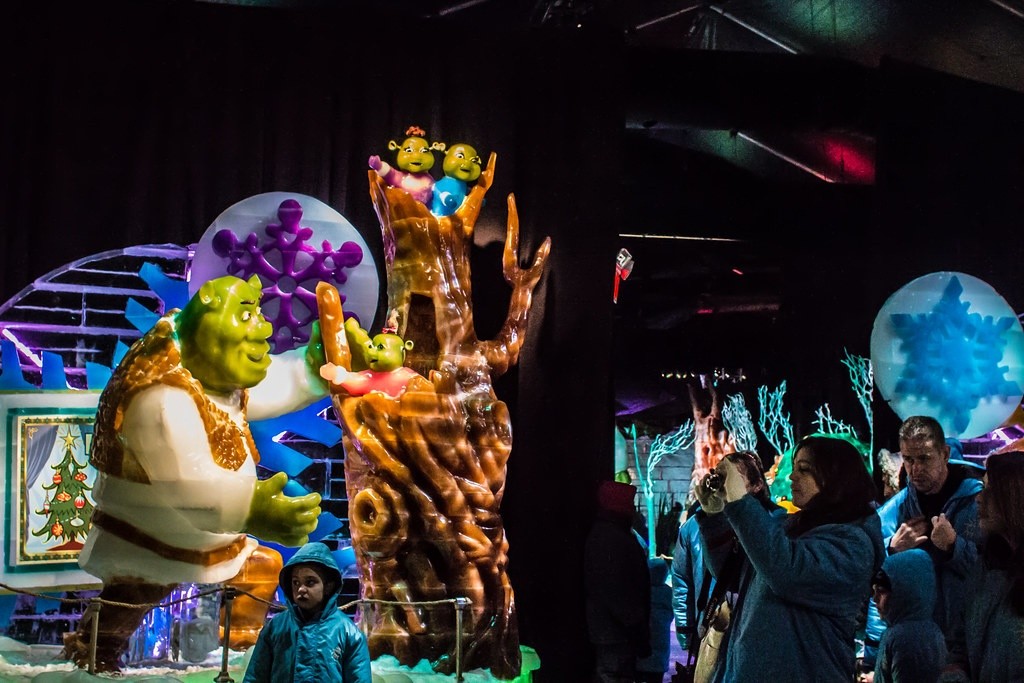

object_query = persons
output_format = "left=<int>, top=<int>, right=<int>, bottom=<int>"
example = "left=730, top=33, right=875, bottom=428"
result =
left=965, top=451, right=1024, bottom=683
left=583, top=480, right=674, bottom=683
left=873, top=549, right=948, bottom=683
left=241, top=542, right=372, bottom=683
left=888, top=416, right=986, bottom=666
left=694, top=437, right=887, bottom=683
left=671, top=449, right=788, bottom=683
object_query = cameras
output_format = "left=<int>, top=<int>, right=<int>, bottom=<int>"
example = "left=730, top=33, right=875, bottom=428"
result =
left=705, top=470, right=725, bottom=491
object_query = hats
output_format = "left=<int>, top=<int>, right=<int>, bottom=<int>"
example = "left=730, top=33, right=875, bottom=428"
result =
left=869, top=570, right=890, bottom=595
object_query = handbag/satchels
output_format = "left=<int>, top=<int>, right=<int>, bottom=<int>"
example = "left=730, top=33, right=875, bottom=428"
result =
left=671, top=635, right=701, bottom=683
